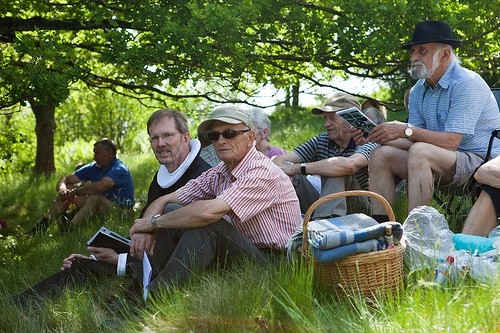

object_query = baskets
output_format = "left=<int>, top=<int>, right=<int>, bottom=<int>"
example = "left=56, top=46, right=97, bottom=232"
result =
left=297, top=188, right=405, bottom=297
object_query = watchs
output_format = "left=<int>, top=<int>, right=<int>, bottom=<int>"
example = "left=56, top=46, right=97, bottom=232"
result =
left=301, top=163, right=306, bottom=175
left=151, top=214, right=164, bottom=228
left=404, top=122, right=415, bottom=141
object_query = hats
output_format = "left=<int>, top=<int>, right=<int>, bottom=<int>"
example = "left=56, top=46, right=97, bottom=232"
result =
left=312, top=92, right=361, bottom=113
left=197, top=106, right=253, bottom=137
left=402, top=20, right=460, bottom=49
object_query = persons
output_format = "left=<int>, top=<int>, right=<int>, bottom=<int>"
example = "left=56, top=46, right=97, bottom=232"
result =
left=12, top=108, right=214, bottom=317
left=103, top=107, right=302, bottom=313
left=245, top=108, right=286, bottom=159
left=27, top=137, right=135, bottom=237
left=347, top=19, right=500, bottom=228
left=460, top=153, right=500, bottom=240
left=270, top=91, right=382, bottom=224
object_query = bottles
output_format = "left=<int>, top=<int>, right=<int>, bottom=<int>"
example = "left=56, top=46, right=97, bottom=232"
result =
left=432, top=256, right=454, bottom=286
left=385, top=225, right=395, bottom=250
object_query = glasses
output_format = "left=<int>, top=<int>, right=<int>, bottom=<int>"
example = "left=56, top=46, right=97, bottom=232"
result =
left=148, top=130, right=184, bottom=140
left=207, top=129, right=253, bottom=140
